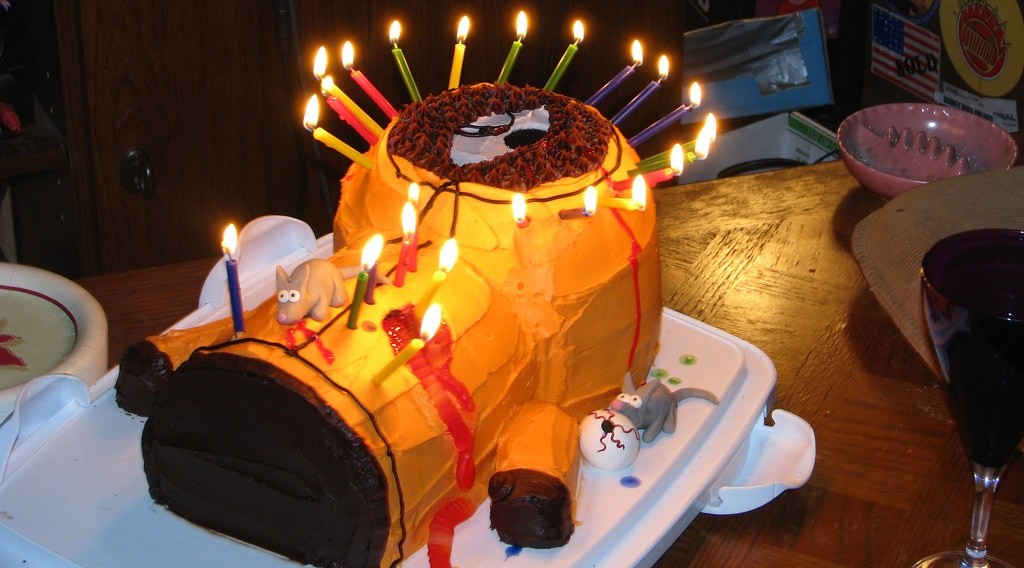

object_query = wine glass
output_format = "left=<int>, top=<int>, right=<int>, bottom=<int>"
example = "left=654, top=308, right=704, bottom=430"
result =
left=908, top=229, right=1023, bottom=568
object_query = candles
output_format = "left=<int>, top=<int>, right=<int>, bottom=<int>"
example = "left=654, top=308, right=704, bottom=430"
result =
left=221, top=12, right=718, bottom=384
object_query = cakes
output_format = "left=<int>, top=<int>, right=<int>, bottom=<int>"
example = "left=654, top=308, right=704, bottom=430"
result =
left=114, top=81, right=723, bottom=568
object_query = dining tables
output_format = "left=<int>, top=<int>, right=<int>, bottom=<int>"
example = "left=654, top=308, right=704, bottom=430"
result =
left=1, top=161, right=1024, bottom=567
